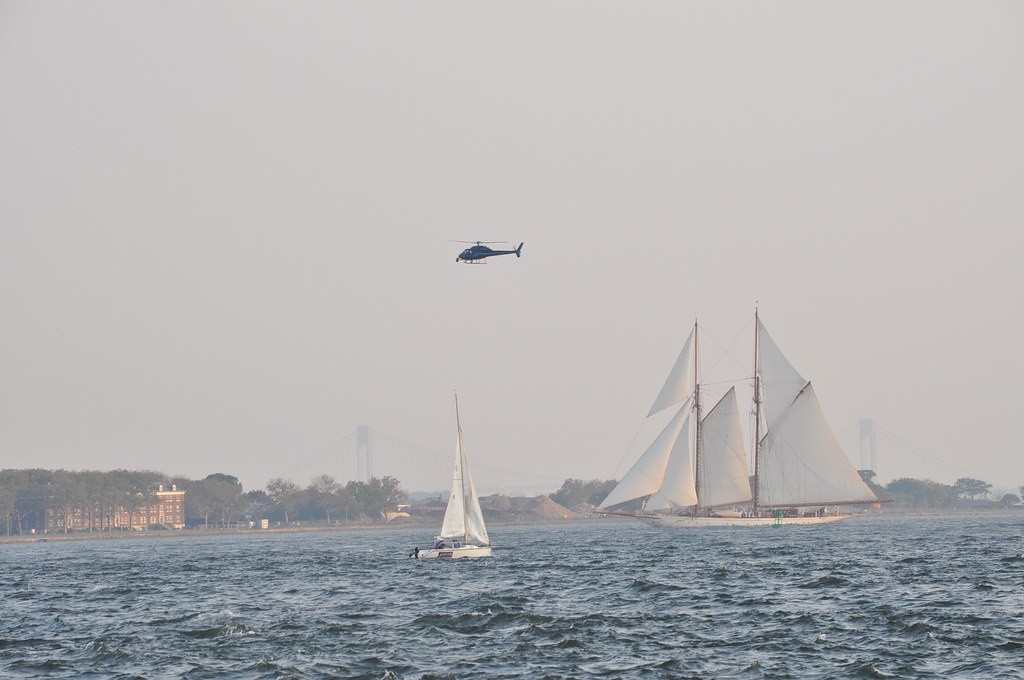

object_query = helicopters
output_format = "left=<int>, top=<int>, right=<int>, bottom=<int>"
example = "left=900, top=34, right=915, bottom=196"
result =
left=448, top=239, right=524, bottom=265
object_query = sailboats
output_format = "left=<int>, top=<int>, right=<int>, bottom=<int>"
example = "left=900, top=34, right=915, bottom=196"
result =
left=593, top=298, right=895, bottom=527
left=409, top=387, right=492, bottom=560
